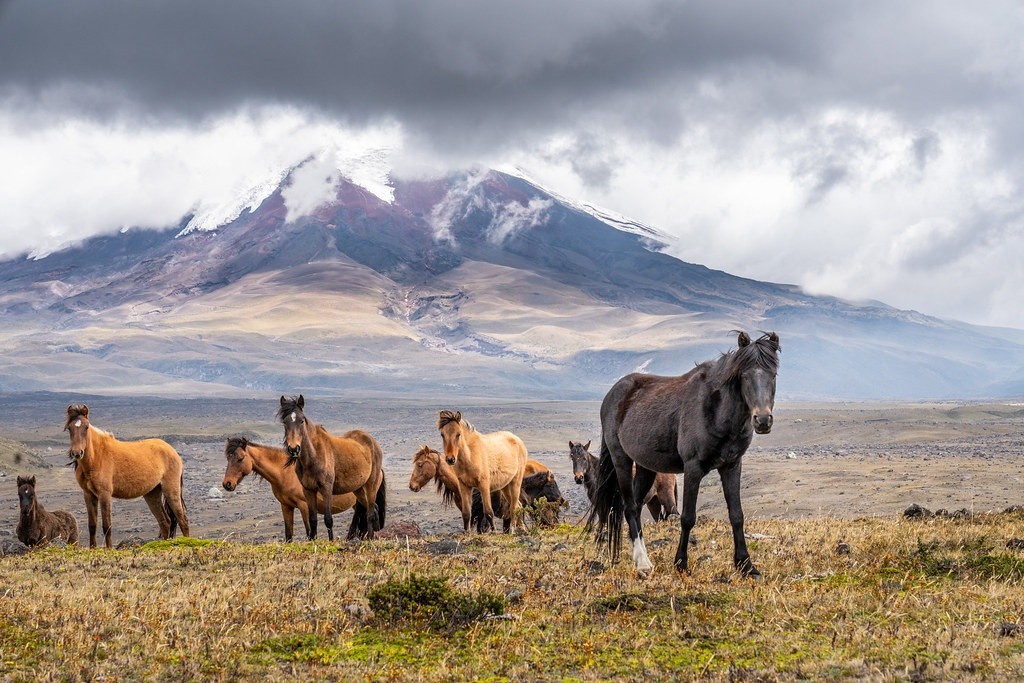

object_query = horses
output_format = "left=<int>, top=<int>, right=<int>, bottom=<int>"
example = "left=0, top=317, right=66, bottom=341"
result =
left=408, top=410, right=565, bottom=534
left=16, top=474, right=78, bottom=544
left=62, top=406, right=191, bottom=548
left=568, top=439, right=680, bottom=520
left=278, top=395, right=381, bottom=540
left=223, top=438, right=385, bottom=544
left=601, top=329, right=780, bottom=580
